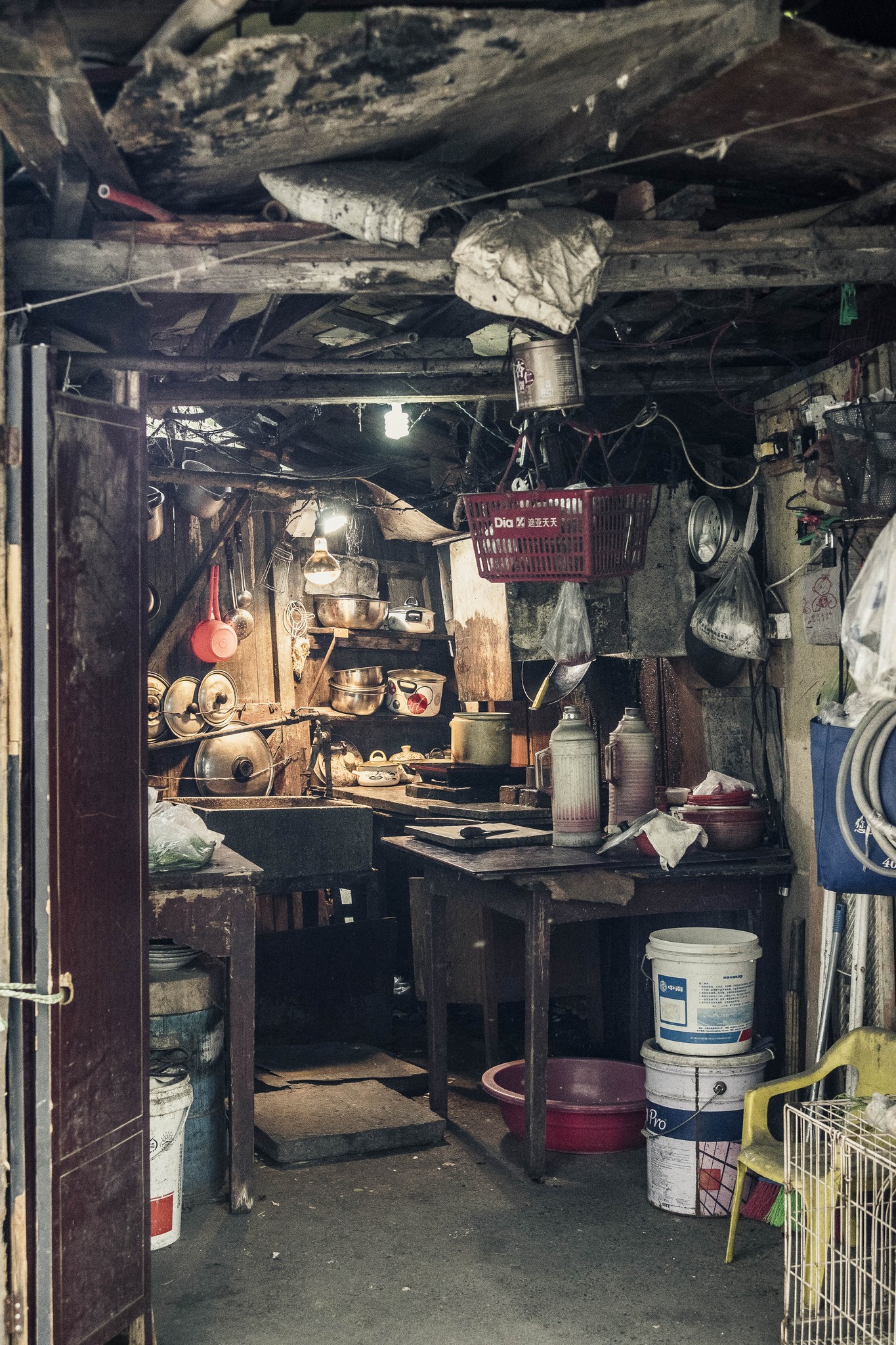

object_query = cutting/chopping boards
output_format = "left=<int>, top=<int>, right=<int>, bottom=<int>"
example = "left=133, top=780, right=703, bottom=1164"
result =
left=403, top=822, right=553, bottom=850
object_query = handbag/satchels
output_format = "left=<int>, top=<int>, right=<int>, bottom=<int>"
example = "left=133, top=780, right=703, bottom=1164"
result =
left=538, top=580, right=597, bottom=667
left=689, top=484, right=769, bottom=661
left=148, top=787, right=225, bottom=874
left=809, top=707, right=895, bottom=896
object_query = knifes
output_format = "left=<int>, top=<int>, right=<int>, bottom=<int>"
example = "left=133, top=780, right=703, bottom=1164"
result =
left=457, top=824, right=518, bottom=839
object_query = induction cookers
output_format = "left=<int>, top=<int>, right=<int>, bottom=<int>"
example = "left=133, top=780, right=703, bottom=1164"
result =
left=410, top=756, right=534, bottom=787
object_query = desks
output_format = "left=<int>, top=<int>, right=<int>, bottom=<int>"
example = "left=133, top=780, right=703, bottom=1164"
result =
left=385, top=831, right=798, bottom=1186
left=149, top=831, right=267, bottom=1220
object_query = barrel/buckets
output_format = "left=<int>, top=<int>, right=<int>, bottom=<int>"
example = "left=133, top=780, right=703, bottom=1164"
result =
left=640, top=924, right=765, bottom=1059
left=148, top=1062, right=198, bottom=1251
left=509, top=315, right=586, bottom=415
left=640, top=1033, right=774, bottom=1218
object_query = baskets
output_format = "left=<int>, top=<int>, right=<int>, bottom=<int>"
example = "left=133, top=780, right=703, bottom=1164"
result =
left=459, top=483, right=654, bottom=583
left=691, top=821, right=766, bottom=851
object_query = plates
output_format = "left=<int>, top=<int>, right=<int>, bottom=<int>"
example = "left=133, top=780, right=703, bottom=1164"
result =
left=148, top=940, right=208, bottom=972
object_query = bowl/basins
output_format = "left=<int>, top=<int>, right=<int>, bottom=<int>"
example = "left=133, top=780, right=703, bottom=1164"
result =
left=483, top=1051, right=647, bottom=1152
left=677, top=787, right=767, bottom=852
left=324, top=664, right=389, bottom=718
left=634, top=826, right=679, bottom=858
left=313, top=594, right=391, bottom=631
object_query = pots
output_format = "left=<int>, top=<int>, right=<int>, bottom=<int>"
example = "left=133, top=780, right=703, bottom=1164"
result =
left=147, top=484, right=166, bottom=545
left=383, top=664, right=448, bottom=717
left=385, top=596, right=436, bottom=634
left=449, top=711, right=515, bottom=766
left=176, top=445, right=238, bottom=521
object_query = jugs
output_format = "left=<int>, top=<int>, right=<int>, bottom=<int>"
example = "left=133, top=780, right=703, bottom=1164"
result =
left=606, top=705, right=657, bottom=834
left=532, top=702, right=603, bottom=850
